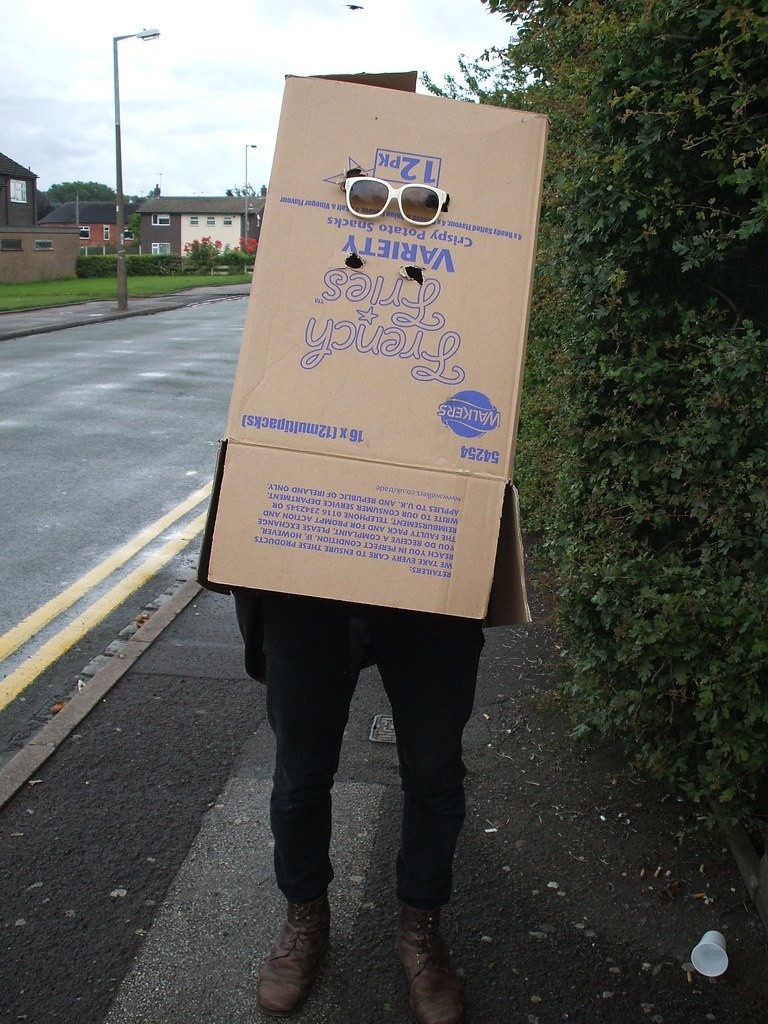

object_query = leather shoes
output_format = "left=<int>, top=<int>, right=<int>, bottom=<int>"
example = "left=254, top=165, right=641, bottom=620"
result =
left=255, top=899, right=330, bottom=1017
left=395, top=902, right=463, bottom=1024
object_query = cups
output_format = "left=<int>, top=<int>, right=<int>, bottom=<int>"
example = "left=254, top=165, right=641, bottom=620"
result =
left=691, top=930, right=729, bottom=977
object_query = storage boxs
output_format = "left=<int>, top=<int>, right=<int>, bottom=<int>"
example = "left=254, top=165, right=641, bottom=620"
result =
left=197, top=66, right=550, bottom=628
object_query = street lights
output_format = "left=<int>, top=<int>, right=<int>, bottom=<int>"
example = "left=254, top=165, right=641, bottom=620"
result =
left=113, top=27, right=160, bottom=313
left=244, top=143, right=258, bottom=275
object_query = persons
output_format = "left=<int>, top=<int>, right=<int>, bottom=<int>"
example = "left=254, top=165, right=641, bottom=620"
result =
left=231, top=587, right=485, bottom=1024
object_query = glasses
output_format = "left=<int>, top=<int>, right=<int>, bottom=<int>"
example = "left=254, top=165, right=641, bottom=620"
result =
left=344, top=175, right=448, bottom=226
